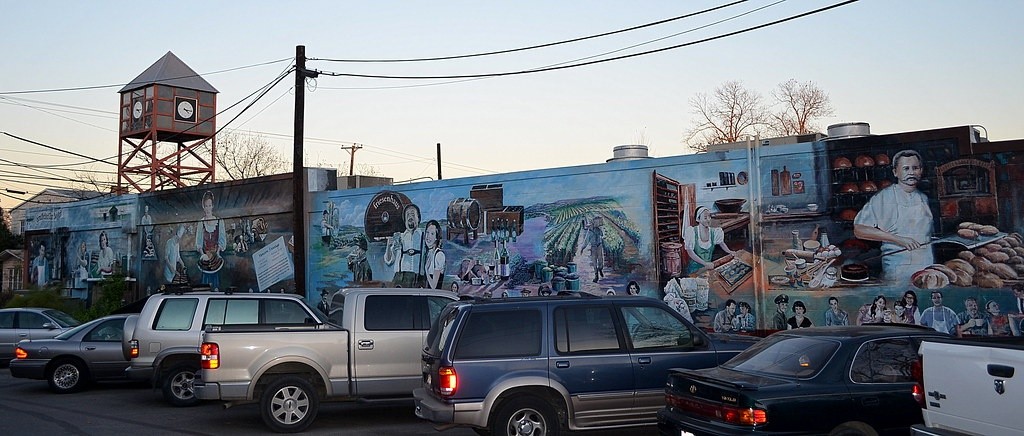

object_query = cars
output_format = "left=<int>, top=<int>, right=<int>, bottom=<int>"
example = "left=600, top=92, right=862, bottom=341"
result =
left=657, top=321, right=950, bottom=436
left=9, top=313, right=140, bottom=394
left=0, top=307, right=84, bottom=364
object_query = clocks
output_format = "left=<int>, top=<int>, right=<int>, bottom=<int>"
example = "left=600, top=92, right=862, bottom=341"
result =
left=133, top=101, right=142, bottom=118
left=178, top=101, right=194, bottom=118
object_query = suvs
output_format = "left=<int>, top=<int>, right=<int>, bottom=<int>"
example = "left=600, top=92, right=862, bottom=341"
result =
left=120, top=287, right=342, bottom=406
left=411, top=290, right=785, bottom=436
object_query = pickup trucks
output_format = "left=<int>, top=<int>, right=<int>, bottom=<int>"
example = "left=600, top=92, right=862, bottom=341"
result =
left=909, top=336, right=1024, bottom=436
left=192, top=286, right=498, bottom=433
